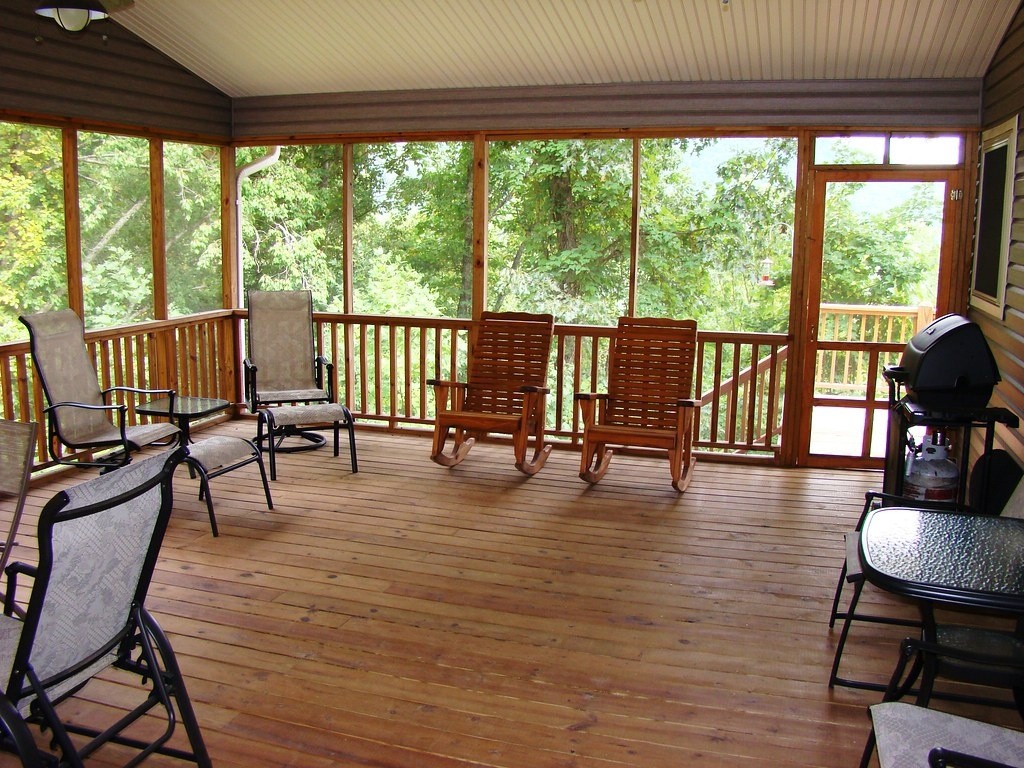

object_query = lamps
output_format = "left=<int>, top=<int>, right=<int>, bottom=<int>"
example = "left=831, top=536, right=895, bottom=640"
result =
left=34, top=0, right=110, bottom=32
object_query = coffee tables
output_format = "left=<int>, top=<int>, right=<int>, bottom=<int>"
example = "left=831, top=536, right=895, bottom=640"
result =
left=859, top=506, right=1024, bottom=721
left=133, top=395, right=231, bottom=479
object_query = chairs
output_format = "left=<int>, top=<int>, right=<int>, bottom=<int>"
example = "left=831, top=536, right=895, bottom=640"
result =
left=0, top=419, right=39, bottom=622
left=243, top=290, right=359, bottom=481
left=828, top=449, right=1024, bottom=689
left=18, top=308, right=273, bottom=537
left=573, top=317, right=703, bottom=492
left=0, top=446, right=213, bottom=768
left=425, top=311, right=555, bottom=475
